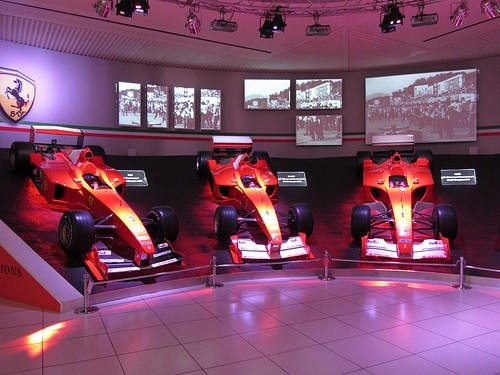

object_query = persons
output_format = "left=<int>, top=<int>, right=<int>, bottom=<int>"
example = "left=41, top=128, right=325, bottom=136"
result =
left=368, top=84, right=475, bottom=139
left=297, top=115, right=342, bottom=141
left=120, top=91, right=220, bottom=130
left=267, top=96, right=290, bottom=109
left=296, top=91, right=342, bottom=109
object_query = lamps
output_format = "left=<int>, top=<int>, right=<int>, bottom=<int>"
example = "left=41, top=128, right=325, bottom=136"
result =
left=378, top=2, right=406, bottom=33
left=185, top=3, right=202, bottom=35
left=258, top=5, right=287, bottom=39
left=93, top=0, right=150, bottom=20
left=449, top=0, right=470, bottom=27
left=479, top=0, right=500, bottom=20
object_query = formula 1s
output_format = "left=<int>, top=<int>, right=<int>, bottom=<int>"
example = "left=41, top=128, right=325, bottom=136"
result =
left=351, top=149, right=457, bottom=263
left=366, top=125, right=422, bottom=144
left=9, top=141, right=186, bottom=282
left=196, top=150, right=315, bottom=266
